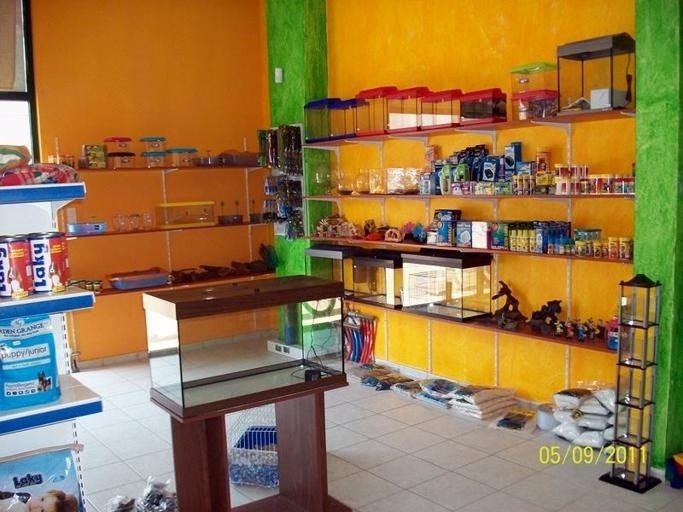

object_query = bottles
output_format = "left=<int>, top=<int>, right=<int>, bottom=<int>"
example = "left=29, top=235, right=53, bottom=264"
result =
left=508, top=219, right=631, bottom=260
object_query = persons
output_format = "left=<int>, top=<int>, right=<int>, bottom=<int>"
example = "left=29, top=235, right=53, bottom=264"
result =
left=0, top=490, right=77, bottom=512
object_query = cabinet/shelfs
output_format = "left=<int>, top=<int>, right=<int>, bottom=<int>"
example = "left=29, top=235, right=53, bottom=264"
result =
left=0, top=182, right=102, bottom=436
left=300, top=109, right=636, bottom=355
left=601, top=275, right=662, bottom=496
left=59, top=165, right=276, bottom=298
